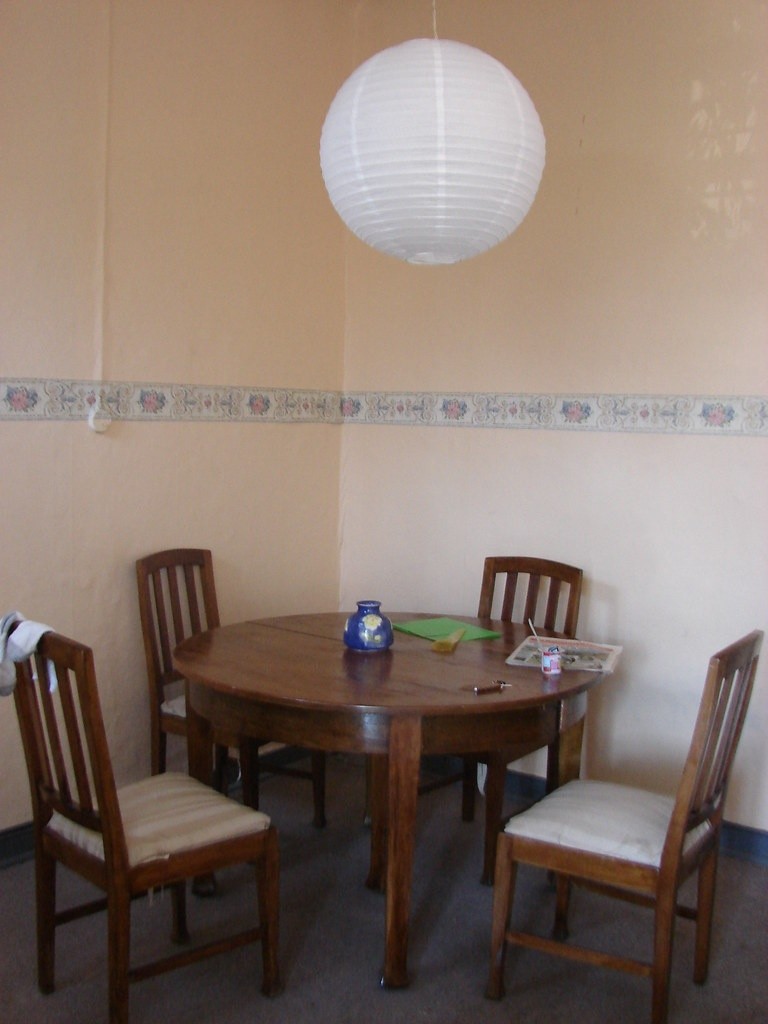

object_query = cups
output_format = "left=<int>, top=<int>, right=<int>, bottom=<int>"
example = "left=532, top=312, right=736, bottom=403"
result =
left=540, top=647, right=565, bottom=673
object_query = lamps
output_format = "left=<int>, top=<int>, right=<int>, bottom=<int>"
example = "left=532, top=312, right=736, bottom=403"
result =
left=319, top=0, right=547, bottom=265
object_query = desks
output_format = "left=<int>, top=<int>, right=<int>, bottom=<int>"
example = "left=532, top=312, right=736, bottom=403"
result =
left=173, top=611, right=620, bottom=990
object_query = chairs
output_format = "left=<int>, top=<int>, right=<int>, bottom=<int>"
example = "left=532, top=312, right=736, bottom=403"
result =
left=484, top=629, right=763, bottom=1024
left=0, top=619, right=286, bottom=1024
left=419, top=556, right=583, bottom=886
left=136, top=549, right=327, bottom=832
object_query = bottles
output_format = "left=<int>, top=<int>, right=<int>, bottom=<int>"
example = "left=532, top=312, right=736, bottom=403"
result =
left=342, top=601, right=393, bottom=651
